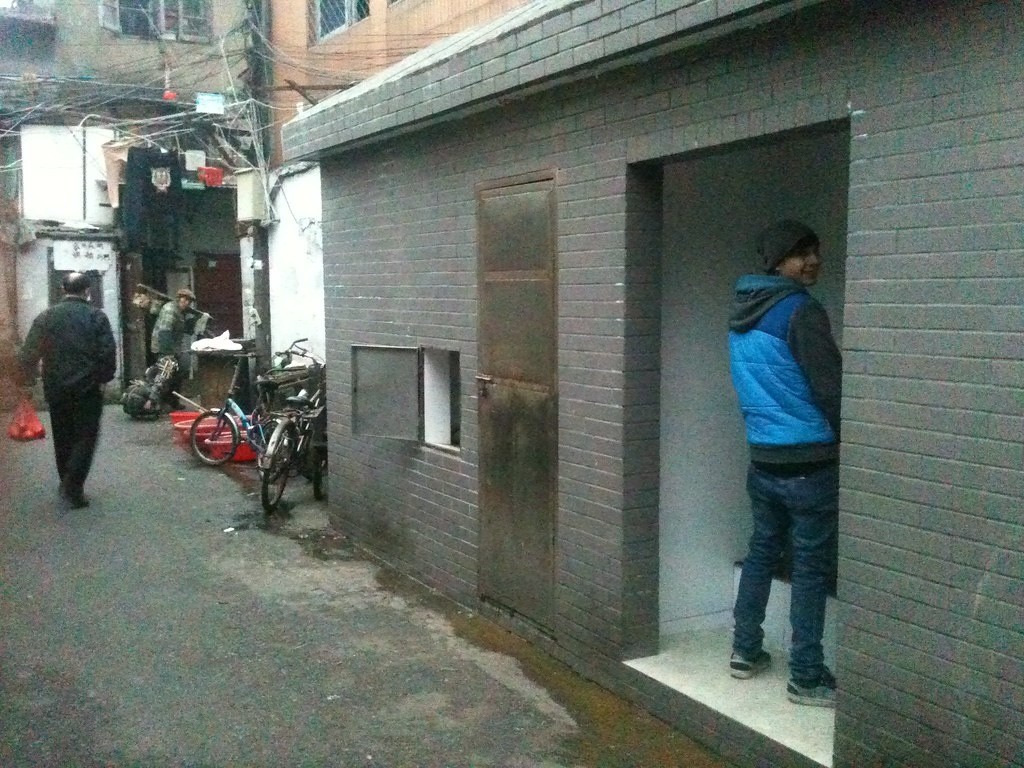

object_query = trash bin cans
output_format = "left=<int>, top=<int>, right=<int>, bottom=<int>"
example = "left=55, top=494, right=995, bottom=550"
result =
left=186, top=340, right=256, bottom=417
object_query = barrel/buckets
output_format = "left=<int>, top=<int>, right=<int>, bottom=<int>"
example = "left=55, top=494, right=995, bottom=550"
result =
left=200, top=356, right=251, bottom=413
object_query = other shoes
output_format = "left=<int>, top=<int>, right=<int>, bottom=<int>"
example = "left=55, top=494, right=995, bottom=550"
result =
left=58, top=472, right=89, bottom=507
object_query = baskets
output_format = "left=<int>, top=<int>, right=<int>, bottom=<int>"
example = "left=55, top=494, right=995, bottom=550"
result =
left=309, top=364, right=327, bottom=404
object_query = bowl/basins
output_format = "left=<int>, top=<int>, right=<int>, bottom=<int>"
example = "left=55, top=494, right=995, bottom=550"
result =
left=170, top=410, right=257, bottom=461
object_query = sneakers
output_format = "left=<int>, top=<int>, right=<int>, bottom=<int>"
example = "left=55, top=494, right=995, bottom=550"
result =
left=787, top=664, right=836, bottom=707
left=730, top=648, right=771, bottom=679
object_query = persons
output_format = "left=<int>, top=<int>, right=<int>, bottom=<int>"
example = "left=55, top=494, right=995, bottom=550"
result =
left=13, top=272, right=118, bottom=509
left=728, top=218, right=842, bottom=709
left=151, top=287, right=197, bottom=410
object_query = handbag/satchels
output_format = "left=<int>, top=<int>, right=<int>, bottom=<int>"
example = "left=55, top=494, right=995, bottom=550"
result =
left=7, top=399, right=45, bottom=441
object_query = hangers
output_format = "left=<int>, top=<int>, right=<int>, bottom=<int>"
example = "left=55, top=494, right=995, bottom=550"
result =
left=102, top=129, right=132, bottom=146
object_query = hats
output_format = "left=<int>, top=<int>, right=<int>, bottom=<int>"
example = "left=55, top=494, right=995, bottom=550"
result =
left=755, top=219, right=819, bottom=275
left=176, top=289, right=196, bottom=301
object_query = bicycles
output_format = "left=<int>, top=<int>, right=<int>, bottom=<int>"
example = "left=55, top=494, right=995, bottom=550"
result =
left=190, top=338, right=327, bottom=511
